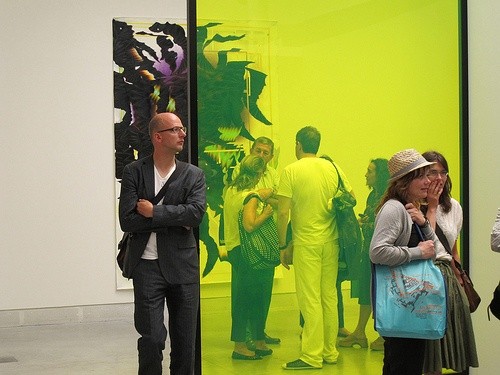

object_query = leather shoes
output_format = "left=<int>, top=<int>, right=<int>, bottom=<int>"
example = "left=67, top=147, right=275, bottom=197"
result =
left=247, top=336, right=257, bottom=350
left=264, top=333, right=280, bottom=343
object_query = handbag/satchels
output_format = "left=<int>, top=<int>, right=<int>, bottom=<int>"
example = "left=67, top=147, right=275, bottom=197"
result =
left=117, top=232, right=135, bottom=274
left=376, top=196, right=447, bottom=340
left=238, top=193, right=281, bottom=271
left=487, top=281, right=500, bottom=321
left=460, top=272, right=481, bottom=313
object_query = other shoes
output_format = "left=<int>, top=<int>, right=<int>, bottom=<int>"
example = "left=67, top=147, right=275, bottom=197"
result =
left=337, top=328, right=352, bottom=337
left=232, top=351, right=260, bottom=360
left=256, top=349, right=273, bottom=356
left=282, top=359, right=316, bottom=370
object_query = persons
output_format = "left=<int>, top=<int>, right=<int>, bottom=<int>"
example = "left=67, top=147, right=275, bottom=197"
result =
left=231, top=136, right=281, bottom=344
left=491, top=208, right=500, bottom=253
left=339, top=159, right=391, bottom=351
left=286, top=155, right=356, bottom=339
left=224, top=155, right=273, bottom=360
left=119, top=112, right=208, bottom=375
left=277, top=126, right=340, bottom=370
left=420, top=151, right=479, bottom=375
left=369, top=149, right=452, bottom=375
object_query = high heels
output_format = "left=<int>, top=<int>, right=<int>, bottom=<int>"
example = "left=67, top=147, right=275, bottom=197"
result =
left=339, top=334, right=368, bottom=348
left=370, top=337, right=385, bottom=351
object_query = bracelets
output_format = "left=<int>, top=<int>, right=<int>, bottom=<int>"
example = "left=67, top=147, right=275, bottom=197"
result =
left=279, top=244, right=287, bottom=250
left=418, top=217, right=430, bottom=228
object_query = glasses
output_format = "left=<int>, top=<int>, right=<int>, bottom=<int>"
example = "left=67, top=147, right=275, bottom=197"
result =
left=430, top=170, right=449, bottom=177
left=157, top=126, right=186, bottom=134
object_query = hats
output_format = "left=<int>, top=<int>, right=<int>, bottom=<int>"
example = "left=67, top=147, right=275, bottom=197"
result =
left=387, top=149, right=438, bottom=183
